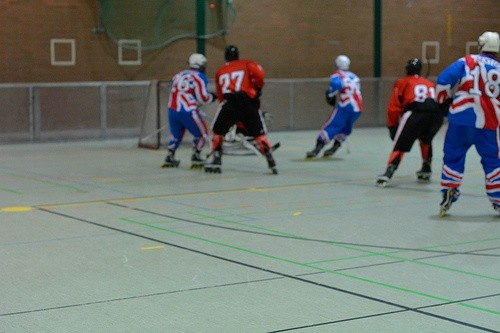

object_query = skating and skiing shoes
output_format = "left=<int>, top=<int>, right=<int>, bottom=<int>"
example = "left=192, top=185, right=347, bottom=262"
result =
left=267, top=154, right=278, bottom=175
left=161, top=152, right=180, bottom=167
left=439, top=188, right=461, bottom=217
left=323, top=147, right=337, bottom=159
left=189, top=152, right=206, bottom=171
left=204, top=156, right=221, bottom=174
left=415, top=170, right=431, bottom=182
left=305, top=149, right=320, bottom=161
left=376, top=170, right=392, bottom=187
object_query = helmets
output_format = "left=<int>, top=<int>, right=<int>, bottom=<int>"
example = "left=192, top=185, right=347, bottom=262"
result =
left=336, top=55, right=351, bottom=71
left=188, top=54, right=206, bottom=70
left=478, top=31, right=500, bottom=53
left=225, top=45, right=239, bottom=61
left=405, top=58, right=422, bottom=74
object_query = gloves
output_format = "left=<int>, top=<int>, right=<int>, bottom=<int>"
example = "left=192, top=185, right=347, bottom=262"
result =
left=210, top=92, right=216, bottom=102
left=326, top=91, right=336, bottom=106
left=440, top=101, right=448, bottom=117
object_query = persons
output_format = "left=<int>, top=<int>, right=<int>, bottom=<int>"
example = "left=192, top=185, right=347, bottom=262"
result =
left=377, top=60, right=445, bottom=189
left=161, top=53, right=217, bottom=170
left=305, top=55, right=363, bottom=160
left=435, top=31, right=500, bottom=216
left=203, top=46, right=278, bottom=175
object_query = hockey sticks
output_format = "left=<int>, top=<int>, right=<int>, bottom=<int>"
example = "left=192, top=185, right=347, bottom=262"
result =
left=202, top=111, right=280, bottom=158
